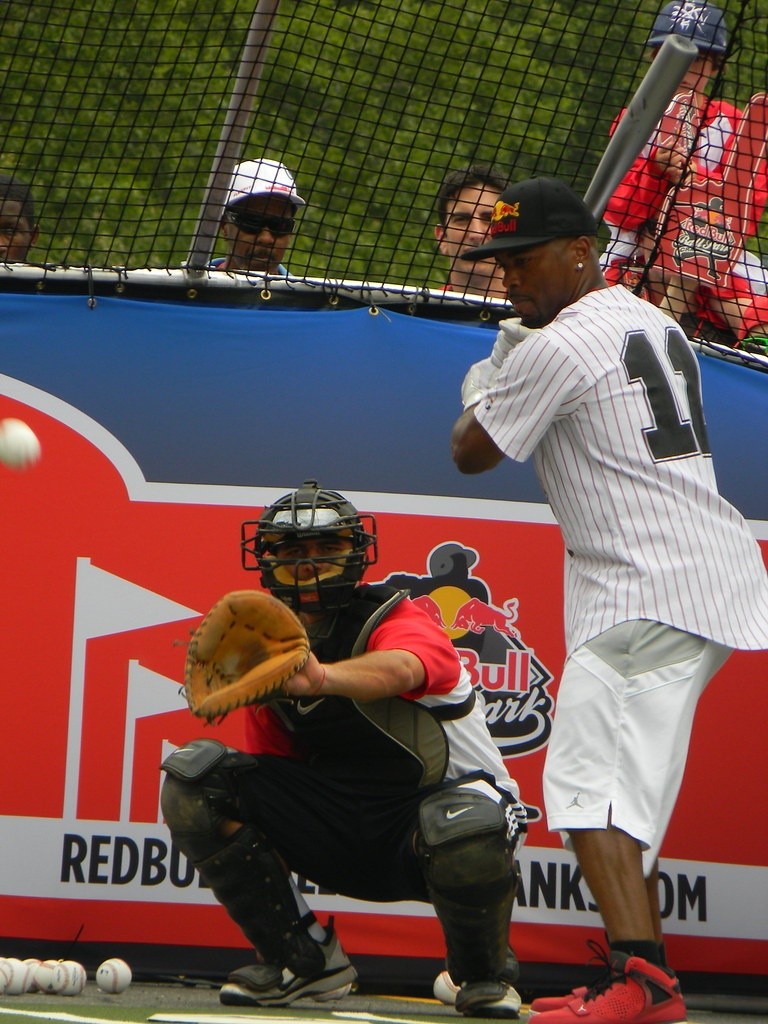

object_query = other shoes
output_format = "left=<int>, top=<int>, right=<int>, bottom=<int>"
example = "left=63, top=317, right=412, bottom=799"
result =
left=733, top=336, right=768, bottom=358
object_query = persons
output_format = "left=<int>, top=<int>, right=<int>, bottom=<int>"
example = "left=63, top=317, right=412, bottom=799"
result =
left=448, top=179, right=767, bottom=1024
left=205, top=158, right=305, bottom=276
left=605, top=181, right=768, bottom=357
left=161, top=480, right=527, bottom=1020
left=0, top=175, right=40, bottom=264
left=598, top=0, right=768, bottom=355
left=434, top=165, right=507, bottom=299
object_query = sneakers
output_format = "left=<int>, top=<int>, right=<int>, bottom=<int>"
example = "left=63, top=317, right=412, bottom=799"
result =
left=219, top=915, right=358, bottom=1007
left=463, top=983, right=522, bottom=1016
left=527, top=939, right=687, bottom=1024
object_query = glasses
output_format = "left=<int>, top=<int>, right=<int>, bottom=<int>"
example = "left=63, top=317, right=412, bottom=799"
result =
left=226, top=207, right=294, bottom=238
left=643, top=218, right=659, bottom=237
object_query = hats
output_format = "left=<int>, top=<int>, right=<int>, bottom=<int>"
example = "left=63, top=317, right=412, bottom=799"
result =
left=460, top=174, right=597, bottom=263
left=221, top=158, right=306, bottom=215
left=644, top=0, right=728, bottom=52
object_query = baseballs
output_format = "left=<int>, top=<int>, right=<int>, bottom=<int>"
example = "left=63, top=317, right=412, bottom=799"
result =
left=96, top=957, right=132, bottom=995
left=52, top=959, right=88, bottom=996
left=432, top=969, right=462, bottom=1005
left=0, top=417, right=43, bottom=466
left=0, top=957, right=59, bottom=996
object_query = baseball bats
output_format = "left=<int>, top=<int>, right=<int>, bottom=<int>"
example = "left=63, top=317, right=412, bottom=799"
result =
left=520, top=33, right=700, bottom=330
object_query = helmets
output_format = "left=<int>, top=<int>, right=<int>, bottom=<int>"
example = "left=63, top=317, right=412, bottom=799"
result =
left=239, top=480, right=379, bottom=617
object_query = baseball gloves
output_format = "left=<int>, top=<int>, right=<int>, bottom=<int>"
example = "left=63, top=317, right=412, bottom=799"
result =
left=183, top=590, right=310, bottom=718
